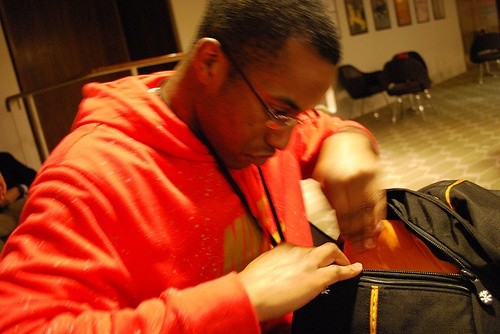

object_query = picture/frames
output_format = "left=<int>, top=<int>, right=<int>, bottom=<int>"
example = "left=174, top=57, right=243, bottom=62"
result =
left=344, top=0, right=368, bottom=36
left=414, top=0, right=430, bottom=24
left=393, top=0, right=411, bottom=27
left=369, top=0, right=391, bottom=31
left=431, top=0, right=446, bottom=20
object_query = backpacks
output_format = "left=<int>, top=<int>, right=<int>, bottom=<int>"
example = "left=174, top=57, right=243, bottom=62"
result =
left=292, top=179, right=500, bottom=334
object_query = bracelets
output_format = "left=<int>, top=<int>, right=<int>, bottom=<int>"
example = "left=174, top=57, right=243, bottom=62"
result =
left=16, top=184, right=24, bottom=200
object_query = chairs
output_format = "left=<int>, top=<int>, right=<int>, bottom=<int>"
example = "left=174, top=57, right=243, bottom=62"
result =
left=470, top=33, right=500, bottom=85
left=338, top=51, right=433, bottom=123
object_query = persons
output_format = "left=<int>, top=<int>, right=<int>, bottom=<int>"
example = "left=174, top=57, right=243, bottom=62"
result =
left=0, top=151, right=38, bottom=244
left=0, top=0, right=386, bottom=334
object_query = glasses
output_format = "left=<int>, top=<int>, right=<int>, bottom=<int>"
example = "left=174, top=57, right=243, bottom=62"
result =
left=224, top=49, right=321, bottom=129
